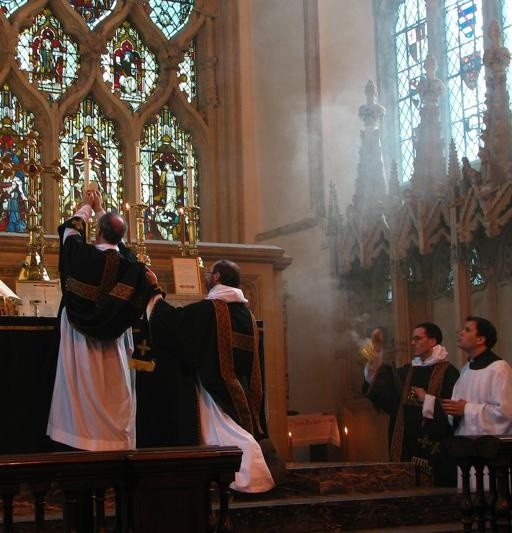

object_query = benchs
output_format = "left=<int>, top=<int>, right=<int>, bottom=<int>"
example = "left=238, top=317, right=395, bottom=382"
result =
left=0, top=446, right=243, bottom=533
left=431, top=435, right=512, bottom=533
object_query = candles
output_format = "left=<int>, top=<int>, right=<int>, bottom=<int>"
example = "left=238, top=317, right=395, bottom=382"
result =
left=289, top=432, right=293, bottom=467
left=83, top=138, right=89, bottom=158
left=188, top=145, right=193, bottom=166
left=345, top=426, right=350, bottom=464
left=27, top=139, right=37, bottom=161
left=135, top=141, right=140, bottom=162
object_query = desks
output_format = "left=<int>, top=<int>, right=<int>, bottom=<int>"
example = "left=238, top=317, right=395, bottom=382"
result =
left=0, top=315, right=267, bottom=473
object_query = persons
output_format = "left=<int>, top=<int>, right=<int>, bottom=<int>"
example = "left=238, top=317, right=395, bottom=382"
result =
left=147, top=259, right=278, bottom=494
left=441, top=316, right=512, bottom=491
left=363, top=322, right=460, bottom=489
left=47, top=189, right=150, bottom=452
